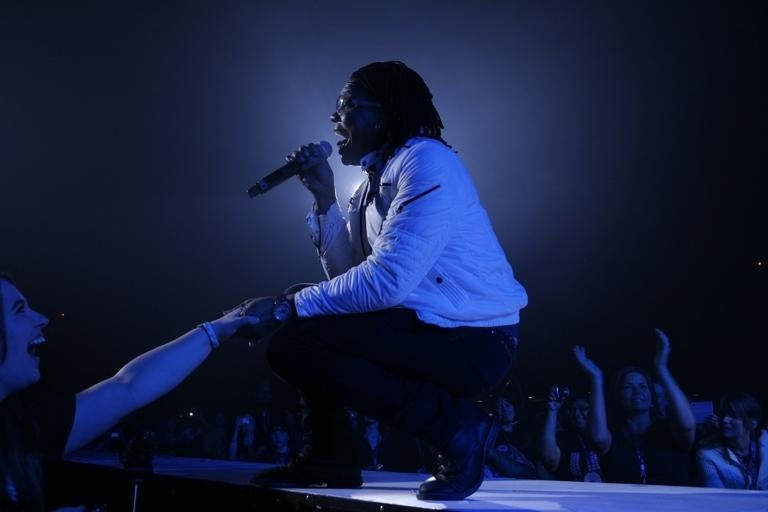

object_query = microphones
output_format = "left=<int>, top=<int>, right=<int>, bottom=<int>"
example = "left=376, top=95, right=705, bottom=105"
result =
left=248, top=140, right=334, bottom=198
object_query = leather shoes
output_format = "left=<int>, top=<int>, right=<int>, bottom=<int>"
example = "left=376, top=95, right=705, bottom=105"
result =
left=249, top=463, right=363, bottom=488
left=417, top=416, right=500, bottom=500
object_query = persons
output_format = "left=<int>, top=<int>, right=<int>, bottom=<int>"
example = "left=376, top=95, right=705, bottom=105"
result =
left=222, top=59, right=530, bottom=499
left=485, top=329, right=766, bottom=491
left=0, top=278, right=260, bottom=512
left=109, top=397, right=436, bottom=475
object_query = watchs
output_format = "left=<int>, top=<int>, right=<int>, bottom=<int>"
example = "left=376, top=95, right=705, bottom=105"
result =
left=273, top=294, right=293, bottom=327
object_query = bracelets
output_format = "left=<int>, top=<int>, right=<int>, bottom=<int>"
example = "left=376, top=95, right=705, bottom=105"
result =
left=198, top=320, right=220, bottom=353
